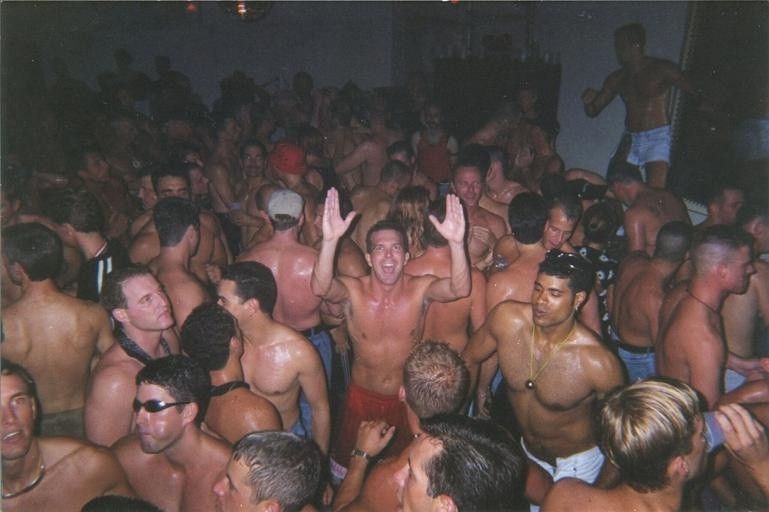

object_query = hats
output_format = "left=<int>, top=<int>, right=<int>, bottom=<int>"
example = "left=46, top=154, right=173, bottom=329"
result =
left=270, top=142, right=306, bottom=175
left=268, top=189, right=305, bottom=224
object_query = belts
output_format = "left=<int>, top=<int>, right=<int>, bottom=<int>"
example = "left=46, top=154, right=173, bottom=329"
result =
left=615, top=340, right=655, bottom=353
left=301, top=325, right=323, bottom=337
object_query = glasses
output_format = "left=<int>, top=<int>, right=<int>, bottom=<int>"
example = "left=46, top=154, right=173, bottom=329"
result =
left=133, top=397, right=190, bottom=412
left=545, top=249, right=588, bottom=293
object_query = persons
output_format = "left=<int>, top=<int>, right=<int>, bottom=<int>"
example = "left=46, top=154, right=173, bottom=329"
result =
left=1, top=24, right=769, bottom=512
